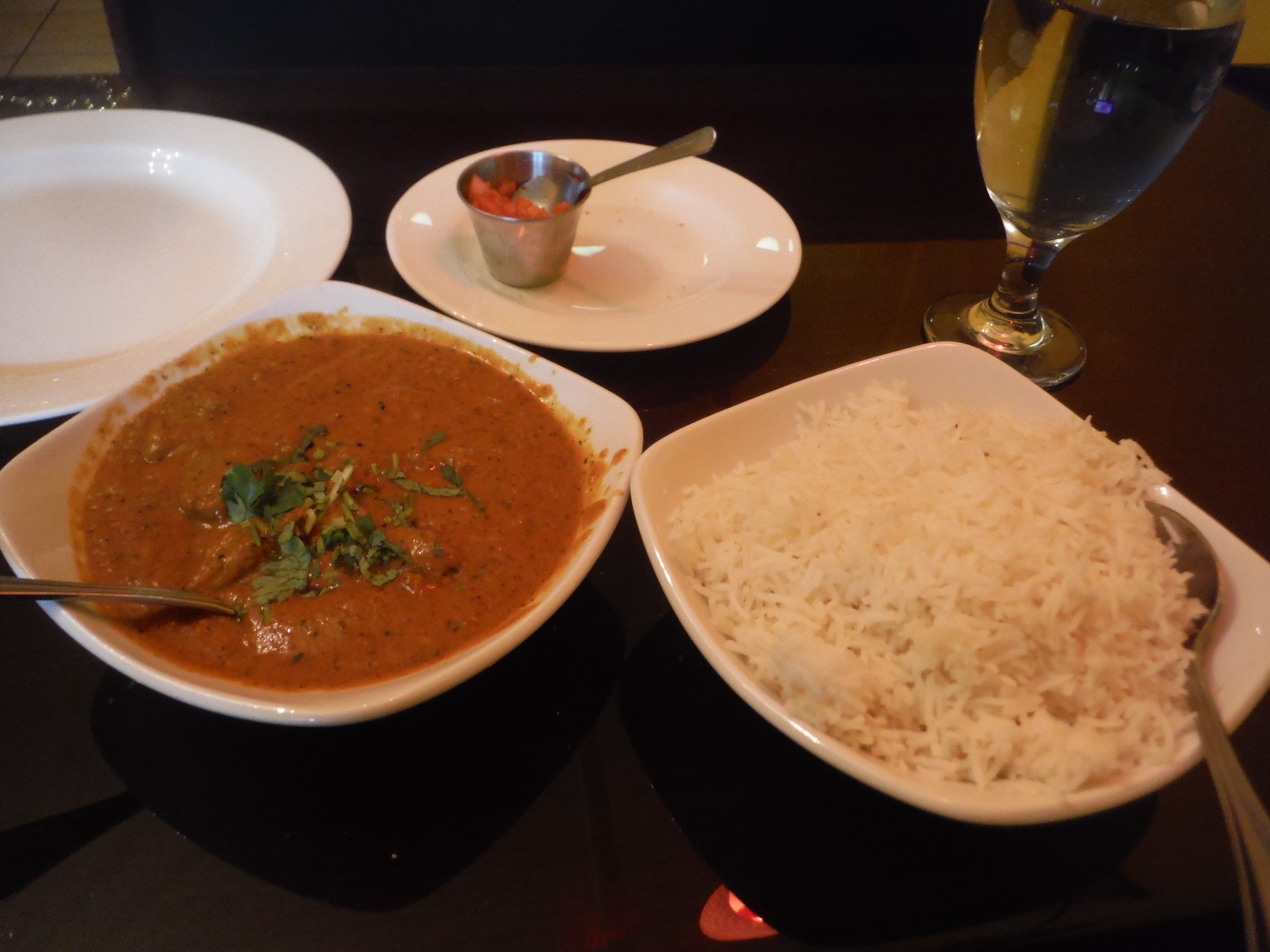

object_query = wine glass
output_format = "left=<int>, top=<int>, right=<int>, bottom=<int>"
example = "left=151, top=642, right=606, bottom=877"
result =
left=923, top=0, right=1249, bottom=388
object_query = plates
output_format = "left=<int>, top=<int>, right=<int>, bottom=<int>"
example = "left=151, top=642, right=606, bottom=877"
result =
left=0, top=110, right=352, bottom=426
left=0, top=280, right=645, bottom=725
left=385, top=138, right=803, bottom=351
left=629, top=340, right=1270, bottom=825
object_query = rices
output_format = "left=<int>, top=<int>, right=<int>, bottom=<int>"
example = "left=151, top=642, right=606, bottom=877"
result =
left=663, top=384, right=1203, bottom=799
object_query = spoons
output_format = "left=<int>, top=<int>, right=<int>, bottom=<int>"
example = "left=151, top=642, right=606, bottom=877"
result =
left=509, top=126, right=717, bottom=210
left=1144, top=501, right=1270, bottom=952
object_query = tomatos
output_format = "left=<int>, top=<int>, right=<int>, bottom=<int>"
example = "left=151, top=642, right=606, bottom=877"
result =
left=468, top=174, right=571, bottom=218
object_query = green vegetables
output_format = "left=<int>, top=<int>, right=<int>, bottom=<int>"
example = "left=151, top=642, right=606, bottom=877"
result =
left=213, top=421, right=487, bottom=664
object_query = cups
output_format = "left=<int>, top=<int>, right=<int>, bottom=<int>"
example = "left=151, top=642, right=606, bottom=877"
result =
left=456, top=150, right=592, bottom=289
